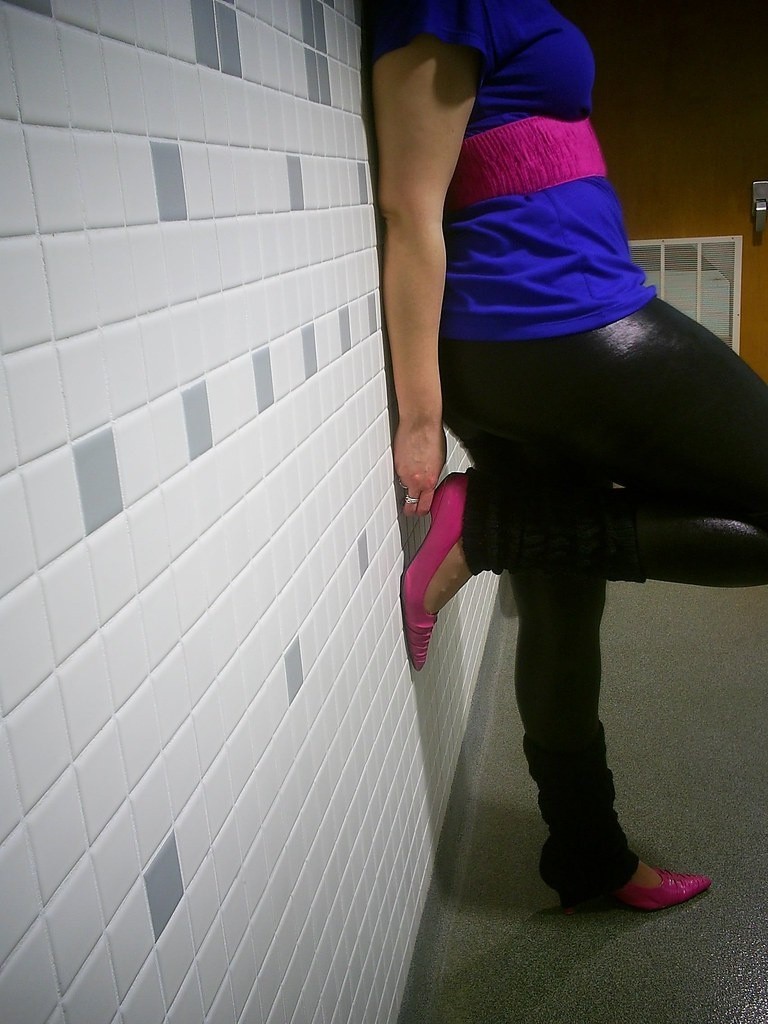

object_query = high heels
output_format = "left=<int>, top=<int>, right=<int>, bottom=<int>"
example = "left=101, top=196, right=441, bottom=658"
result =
left=539, top=849, right=714, bottom=917
left=400, top=471, right=470, bottom=672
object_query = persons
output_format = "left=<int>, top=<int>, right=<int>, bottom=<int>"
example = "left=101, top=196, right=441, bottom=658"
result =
left=361, top=0, right=767, bottom=917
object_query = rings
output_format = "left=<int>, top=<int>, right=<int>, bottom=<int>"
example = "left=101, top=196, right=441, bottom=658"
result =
left=404, top=495, right=419, bottom=504
left=399, top=479, right=407, bottom=489
